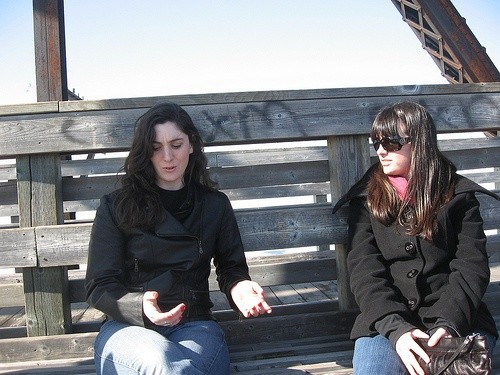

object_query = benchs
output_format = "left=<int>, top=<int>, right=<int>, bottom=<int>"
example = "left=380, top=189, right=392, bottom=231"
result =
left=0, top=79, right=500, bottom=375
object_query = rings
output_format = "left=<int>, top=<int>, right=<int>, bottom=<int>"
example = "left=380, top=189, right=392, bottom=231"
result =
left=163, top=321, right=170, bottom=326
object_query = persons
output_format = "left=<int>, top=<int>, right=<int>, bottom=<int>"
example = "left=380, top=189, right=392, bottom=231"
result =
left=86, top=104, right=271, bottom=375
left=332, top=103, right=499, bottom=375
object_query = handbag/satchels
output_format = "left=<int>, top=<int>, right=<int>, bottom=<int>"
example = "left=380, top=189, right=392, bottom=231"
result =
left=408, top=334, right=493, bottom=375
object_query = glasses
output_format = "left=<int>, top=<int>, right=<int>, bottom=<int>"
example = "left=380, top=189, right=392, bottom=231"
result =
left=370, top=136, right=413, bottom=153
